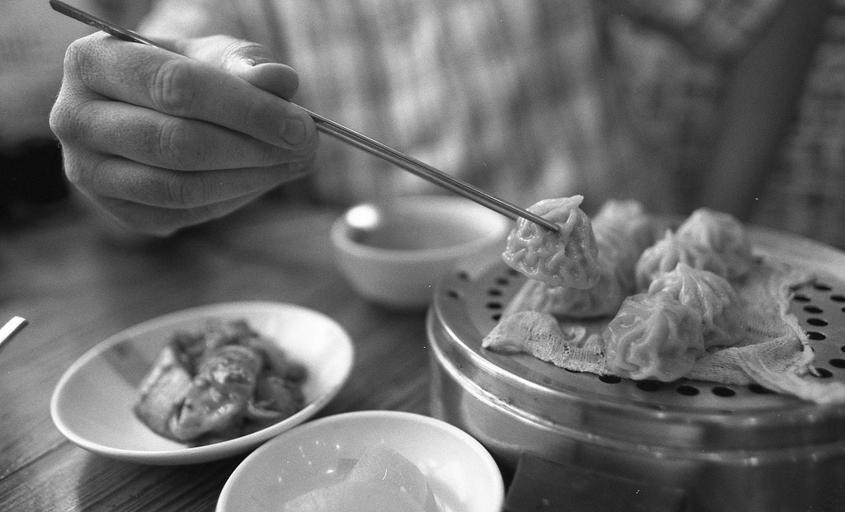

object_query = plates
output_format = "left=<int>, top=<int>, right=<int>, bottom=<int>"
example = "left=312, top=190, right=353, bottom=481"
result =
left=45, top=298, right=355, bottom=470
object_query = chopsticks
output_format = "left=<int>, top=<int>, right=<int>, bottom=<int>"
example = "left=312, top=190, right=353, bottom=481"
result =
left=47, top=0, right=567, bottom=236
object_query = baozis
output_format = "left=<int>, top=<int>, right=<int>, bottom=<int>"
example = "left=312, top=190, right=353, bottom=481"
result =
left=502, top=194, right=750, bottom=385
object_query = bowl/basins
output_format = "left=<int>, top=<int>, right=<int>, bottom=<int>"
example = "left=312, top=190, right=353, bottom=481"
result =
left=211, top=412, right=506, bottom=512
left=329, top=199, right=510, bottom=312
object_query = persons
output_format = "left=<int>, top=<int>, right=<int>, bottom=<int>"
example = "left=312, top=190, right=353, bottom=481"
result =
left=45, top=1, right=845, bottom=250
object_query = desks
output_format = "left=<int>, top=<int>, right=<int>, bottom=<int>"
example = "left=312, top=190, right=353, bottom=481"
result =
left=0, top=189, right=432, bottom=511
